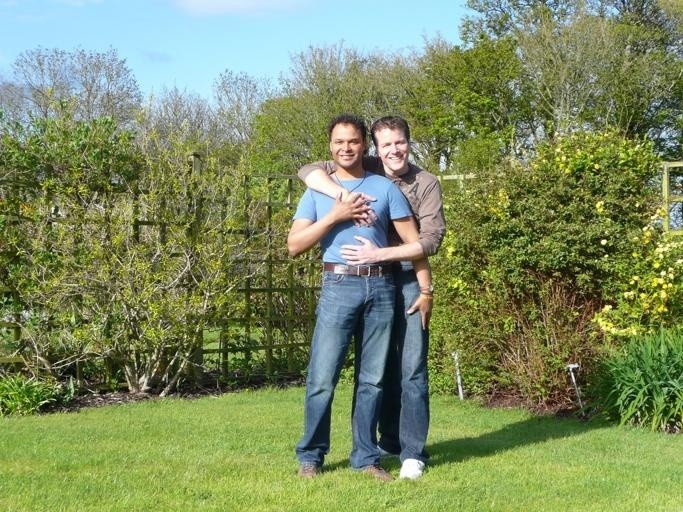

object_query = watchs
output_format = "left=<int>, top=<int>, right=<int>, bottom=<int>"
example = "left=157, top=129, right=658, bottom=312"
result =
left=419, top=285, right=434, bottom=292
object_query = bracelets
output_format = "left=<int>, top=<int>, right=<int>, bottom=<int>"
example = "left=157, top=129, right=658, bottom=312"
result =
left=420, top=292, right=434, bottom=300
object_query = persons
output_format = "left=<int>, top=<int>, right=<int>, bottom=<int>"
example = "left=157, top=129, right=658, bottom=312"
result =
left=284, top=112, right=437, bottom=482
left=298, top=116, right=450, bottom=480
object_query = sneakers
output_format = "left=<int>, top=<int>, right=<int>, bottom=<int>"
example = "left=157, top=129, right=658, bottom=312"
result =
left=377, top=445, right=399, bottom=458
left=363, top=464, right=394, bottom=482
left=298, top=464, right=322, bottom=478
left=399, top=457, right=426, bottom=480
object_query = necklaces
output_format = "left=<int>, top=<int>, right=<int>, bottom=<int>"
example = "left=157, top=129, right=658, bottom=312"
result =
left=334, top=169, right=368, bottom=192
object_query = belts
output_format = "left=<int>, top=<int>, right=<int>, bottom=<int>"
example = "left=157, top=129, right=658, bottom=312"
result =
left=323, top=262, right=390, bottom=276
